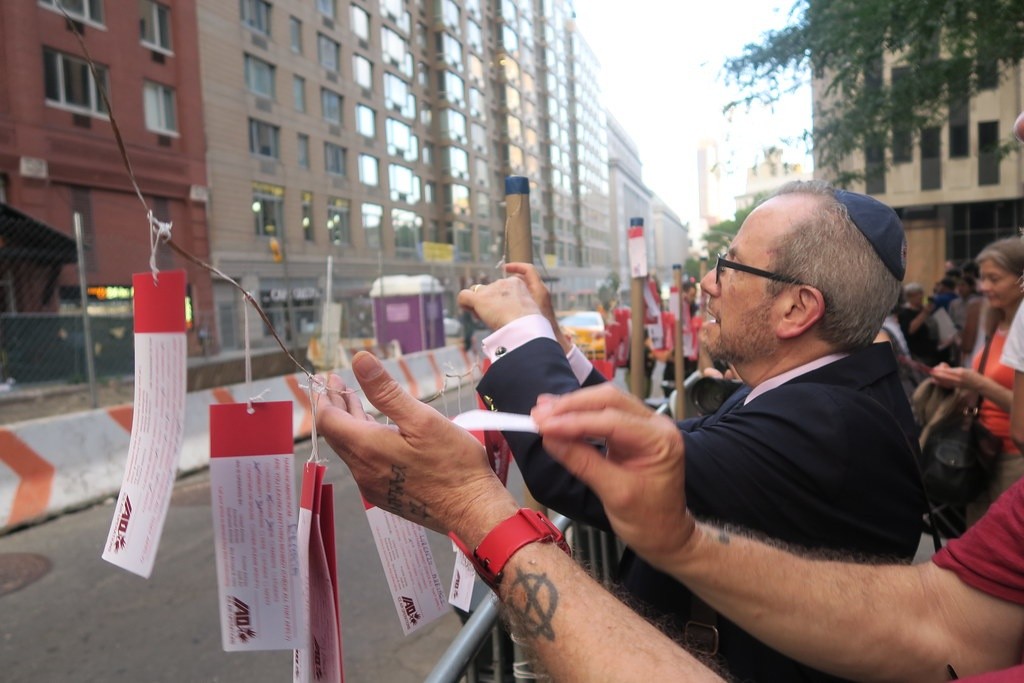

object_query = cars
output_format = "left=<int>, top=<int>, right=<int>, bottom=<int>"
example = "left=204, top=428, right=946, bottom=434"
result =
left=560, top=312, right=605, bottom=360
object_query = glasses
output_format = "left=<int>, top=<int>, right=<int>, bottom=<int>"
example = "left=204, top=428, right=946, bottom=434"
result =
left=715, top=253, right=803, bottom=284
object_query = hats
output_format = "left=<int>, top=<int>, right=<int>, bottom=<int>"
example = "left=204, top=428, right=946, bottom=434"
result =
left=831, top=188, right=908, bottom=281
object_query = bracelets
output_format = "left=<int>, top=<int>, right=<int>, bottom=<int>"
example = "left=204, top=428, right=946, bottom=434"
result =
left=472, top=508, right=572, bottom=602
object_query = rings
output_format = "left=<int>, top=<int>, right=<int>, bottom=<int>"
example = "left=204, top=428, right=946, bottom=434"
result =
left=474, top=284, right=480, bottom=292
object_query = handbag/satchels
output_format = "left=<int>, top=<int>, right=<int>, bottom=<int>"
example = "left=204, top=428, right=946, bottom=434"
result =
left=923, top=429, right=984, bottom=505
left=926, top=307, right=958, bottom=352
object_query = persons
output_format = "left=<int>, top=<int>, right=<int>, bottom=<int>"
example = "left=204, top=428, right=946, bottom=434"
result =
left=312, top=350, right=1024, bottom=683
left=874, top=257, right=982, bottom=394
left=697, top=320, right=740, bottom=380
left=999, top=299, right=1024, bottom=453
left=623, top=326, right=656, bottom=397
left=457, top=181, right=927, bottom=683
left=662, top=283, right=697, bottom=398
left=932, top=236, right=1024, bottom=531
